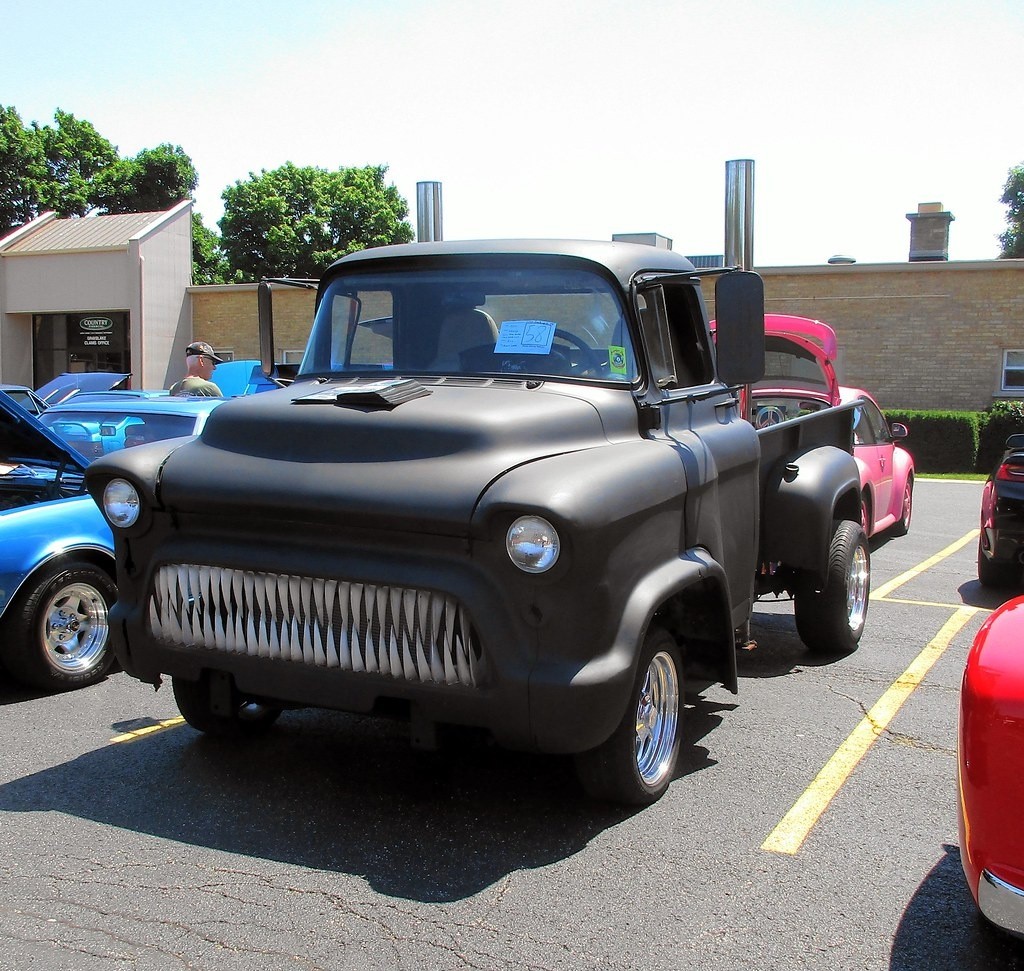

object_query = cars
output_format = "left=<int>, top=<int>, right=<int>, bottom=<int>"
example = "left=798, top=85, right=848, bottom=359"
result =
left=977, top=443, right=1024, bottom=597
left=709, top=313, right=915, bottom=542
left=0, top=358, right=395, bottom=696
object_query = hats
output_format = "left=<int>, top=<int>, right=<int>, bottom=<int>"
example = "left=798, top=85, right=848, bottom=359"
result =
left=186, top=342, right=223, bottom=365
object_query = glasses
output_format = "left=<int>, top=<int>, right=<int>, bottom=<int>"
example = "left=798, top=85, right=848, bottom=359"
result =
left=197, top=356, right=216, bottom=366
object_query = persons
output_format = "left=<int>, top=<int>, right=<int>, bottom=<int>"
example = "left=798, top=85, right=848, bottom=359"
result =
left=170, top=342, right=224, bottom=398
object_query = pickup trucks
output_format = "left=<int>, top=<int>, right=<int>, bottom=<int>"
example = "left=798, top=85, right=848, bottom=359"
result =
left=83, top=164, right=871, bottom=810
